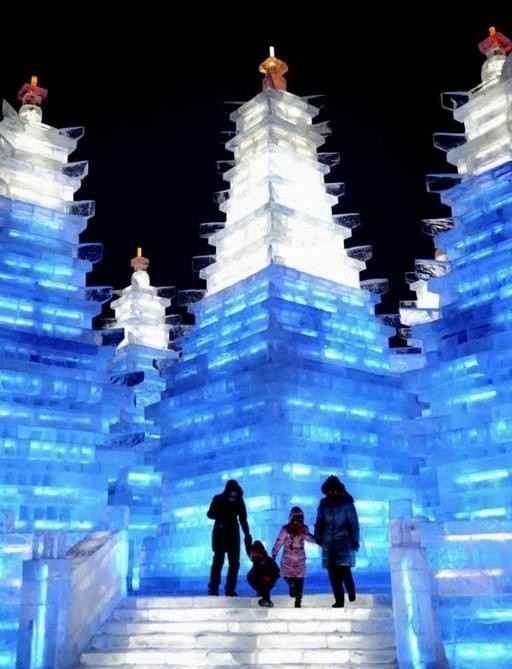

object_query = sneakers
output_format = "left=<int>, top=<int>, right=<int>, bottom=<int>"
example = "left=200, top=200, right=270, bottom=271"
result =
left=207, top=586, right=237, bottom=596
left=259, top=599, right=301, bottom=608
left=332, top=594, right=356, bottom=607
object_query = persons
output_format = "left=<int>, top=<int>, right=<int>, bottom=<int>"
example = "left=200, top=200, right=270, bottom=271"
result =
left=313, top=476, right=360, bottom=607
left=207, top=480, right=249, bottom=596
left=272, top=507, right=316, bottom=608
left=244, top=535, right=279, bottom=607
